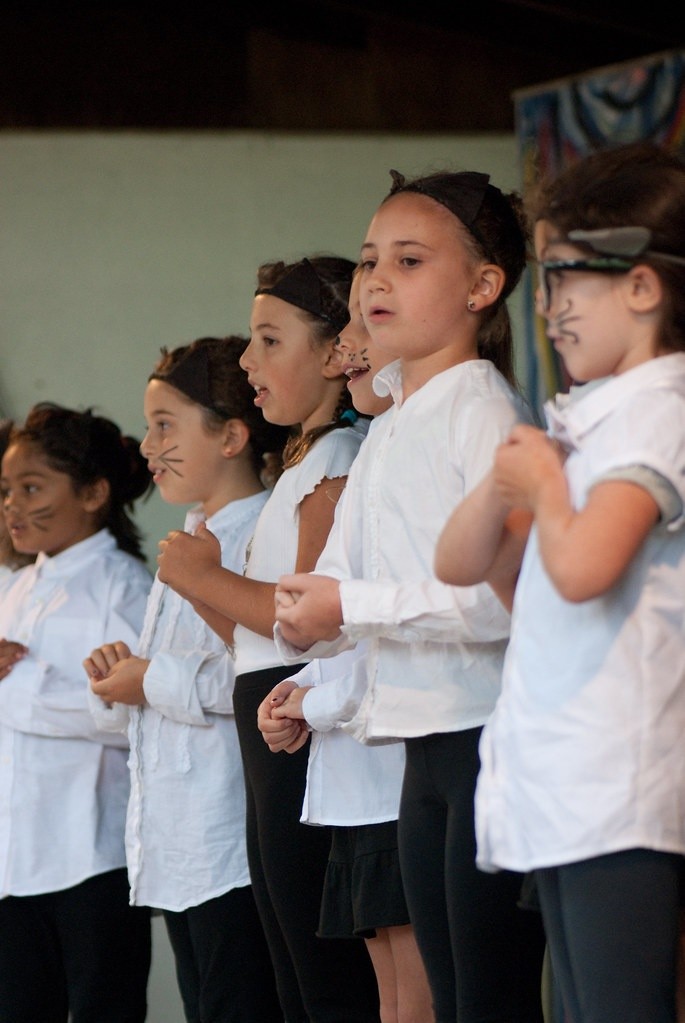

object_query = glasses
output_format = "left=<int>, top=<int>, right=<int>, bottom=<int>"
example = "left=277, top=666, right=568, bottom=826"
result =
left=536, top=258, right=632, bottom=310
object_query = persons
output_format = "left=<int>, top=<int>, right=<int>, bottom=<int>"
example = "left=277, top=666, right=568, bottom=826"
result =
left=84, top=337, right=290, bottom=1023
left=0, top=402, right=155, bottom=1023
left=155, top=257, right=356, bottom=1023
left=274, top=172, right=540, bottom=1023
left=257, top=258, right=512, bottom=1023
left=434, top=146, right=685, bottom=1023
